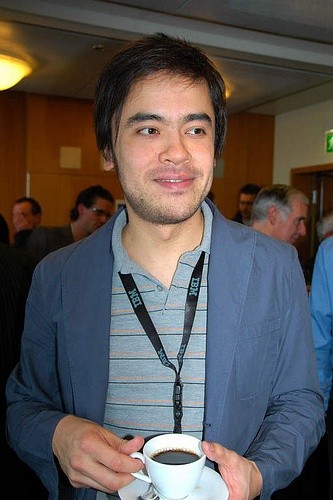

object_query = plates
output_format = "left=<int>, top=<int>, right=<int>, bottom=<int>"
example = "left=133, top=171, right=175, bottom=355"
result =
left=117, top=463, right=230, bottom=500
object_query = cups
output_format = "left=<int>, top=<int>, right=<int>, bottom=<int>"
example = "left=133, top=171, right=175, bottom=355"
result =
left=127, top=433, right=206, bottom=499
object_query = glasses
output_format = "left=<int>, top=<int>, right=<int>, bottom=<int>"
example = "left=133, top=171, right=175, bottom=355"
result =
left=85, top=206, right=112, bottom=219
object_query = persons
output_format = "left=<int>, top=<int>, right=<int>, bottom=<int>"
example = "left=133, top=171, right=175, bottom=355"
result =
left=248, top=184, right=310, bottom=246
left=8, top=185, right=116, bottom=337
left=10, top=197, right=43, bottom=232
left=6, top=34, right=326, bottom=500
left=230, top=184, right=262, bottom=227
left=307, top=236, right=332, bottom=411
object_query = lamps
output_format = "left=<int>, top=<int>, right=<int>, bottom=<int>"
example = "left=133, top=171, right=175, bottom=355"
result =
left=0, top=54, right=32, bottom=91
